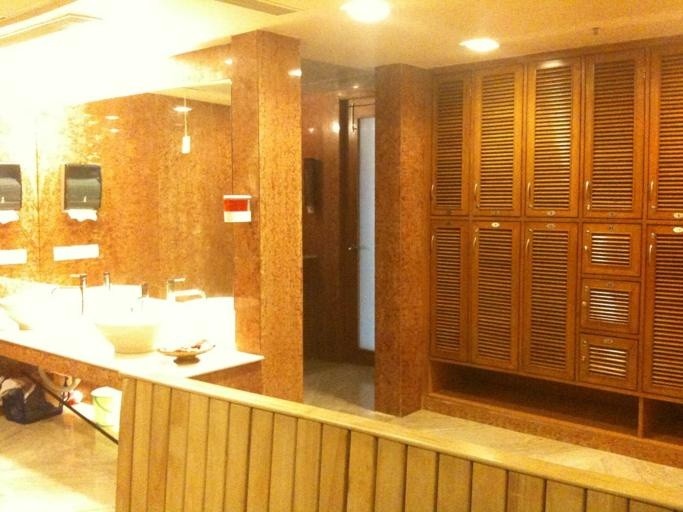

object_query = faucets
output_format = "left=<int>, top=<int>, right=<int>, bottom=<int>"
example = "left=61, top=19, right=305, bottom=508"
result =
left=166, top=278, right=207, bottom=306
left=52, top=273, right=87, bottom=312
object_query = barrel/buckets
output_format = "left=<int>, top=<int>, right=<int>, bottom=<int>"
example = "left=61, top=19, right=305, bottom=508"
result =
left=91, top=387, right=117, bottom=427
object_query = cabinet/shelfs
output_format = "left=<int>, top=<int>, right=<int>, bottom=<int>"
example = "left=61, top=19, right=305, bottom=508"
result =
left=428, top=40, right=682, bottom=409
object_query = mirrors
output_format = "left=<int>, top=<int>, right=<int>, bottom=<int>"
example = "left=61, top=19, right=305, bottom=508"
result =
left=0, top=44, right=238, bottom=355
